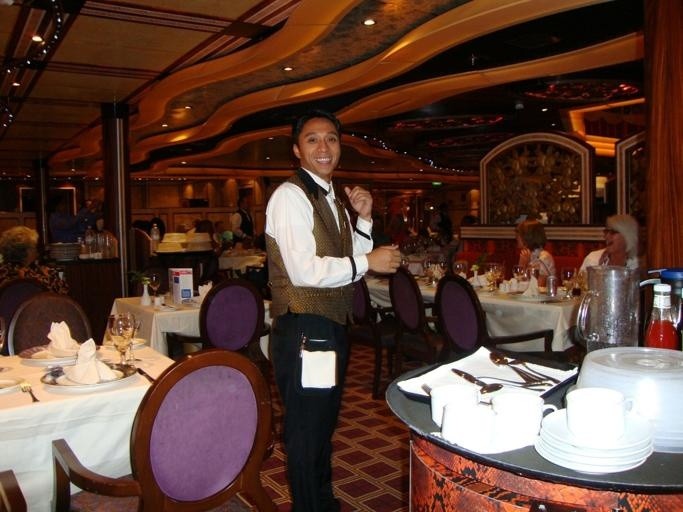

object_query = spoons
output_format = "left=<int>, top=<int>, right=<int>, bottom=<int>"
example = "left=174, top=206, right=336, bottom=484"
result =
left=509, top=356, right=562, bottom=385
left=490, top=351, right=536, bottom=384
left=451, top=368, right=503, bottom=394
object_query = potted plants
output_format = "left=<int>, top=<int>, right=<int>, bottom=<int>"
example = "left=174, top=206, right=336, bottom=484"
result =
left=126, top=270, right=151, bottom=305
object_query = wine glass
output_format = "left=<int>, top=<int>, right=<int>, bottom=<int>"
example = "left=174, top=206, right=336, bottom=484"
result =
left=0, top=316, right=6, bottom=354
left=148, top=272, right=161, bottom=301
left=118, top=312, right=137, bottom=369
left=400, top=256, right=577, bottom=299
left=108, top=314, right=128, bottom=366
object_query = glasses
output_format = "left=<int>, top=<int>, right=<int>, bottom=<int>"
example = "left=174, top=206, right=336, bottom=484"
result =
left=603, top=229, right=620, bottom=235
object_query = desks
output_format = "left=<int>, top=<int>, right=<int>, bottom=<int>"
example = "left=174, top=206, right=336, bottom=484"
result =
left=384, top=355, right=683, bottom=512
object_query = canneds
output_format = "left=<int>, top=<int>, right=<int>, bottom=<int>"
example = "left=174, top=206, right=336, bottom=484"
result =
left=546, top=276, right=557, bottom=297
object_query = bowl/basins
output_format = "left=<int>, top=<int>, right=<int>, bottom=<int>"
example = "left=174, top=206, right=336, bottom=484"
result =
left=564, top=346, right=683, bottom=454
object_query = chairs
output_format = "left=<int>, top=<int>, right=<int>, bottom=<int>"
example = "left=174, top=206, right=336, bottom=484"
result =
left=435, top=274, right=553, bottom=359
left=0, top=470, right=28, bottom=512
left=7, top=291, right=92, bottom=355
left=0, top=278, right=46, bottom=324
left=167, top=279, right=270, bottom=361
left=389, top=265, right=438, bottom=384
left=51, top=348, right=273, bottom=512
left=348, top=276, right=399, bottom=400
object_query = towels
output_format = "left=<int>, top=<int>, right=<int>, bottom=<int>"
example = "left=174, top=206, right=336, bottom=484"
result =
left=55, top=338, right=117, bottom=387
left=509, top=277, right=526, bottom=292
left=32, top=321, right=80, bottom=357
left=198, top=281, right=212, bottom=302
left=301, top=350, right=337, bottom=389
left=523, top=275, right=538, bottom=296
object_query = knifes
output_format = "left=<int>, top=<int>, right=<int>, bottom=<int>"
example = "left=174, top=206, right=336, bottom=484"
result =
left=137, top=367, right=156, bottom=385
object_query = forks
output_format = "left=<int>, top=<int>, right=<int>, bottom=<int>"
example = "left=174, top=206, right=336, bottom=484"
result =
left=20, top=384, right=40, bottom=403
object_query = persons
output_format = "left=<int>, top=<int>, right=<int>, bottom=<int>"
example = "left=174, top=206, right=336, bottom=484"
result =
left=579, top=215, right=639, bottom=274
left=514, top=219, right=555, bottom=290
left=50, top=201, right=72, bottom=240
left=433, top=204, right=450, bottom=247
left=93, top=217, right=107, bottom=232
left=417, top=218, right=429, bottom=236
left=210, top=220, right=226, bottom=243
left=232, top=196, right=254, bottom=245
left=264, top=111, right=401, bottom=511
left=0, top=225, right=69, bottom=351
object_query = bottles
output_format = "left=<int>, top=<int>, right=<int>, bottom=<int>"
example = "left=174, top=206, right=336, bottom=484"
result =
left=661, top=269, right=683, bottom=350
left=645, top=285, right=679, bottom=350
left=151, top=224, right=160, bottom=250
left=77, top=226, right=113, bottom=259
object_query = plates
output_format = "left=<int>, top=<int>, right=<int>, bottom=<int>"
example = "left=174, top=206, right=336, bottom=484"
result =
left=534, top=408, right=655, bottom=476
left=0, top=375, right=26, bottom=393
left=18, top=346, right=100, bottom=367
left=41, top=364, right=137, bottom=394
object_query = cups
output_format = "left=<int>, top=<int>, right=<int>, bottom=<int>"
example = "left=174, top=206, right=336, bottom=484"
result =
left=430, top=385, right=481, bottom=427
left=491, top=392, right=557, bottom=429
left=575, top=265, right=641, bottom=355
